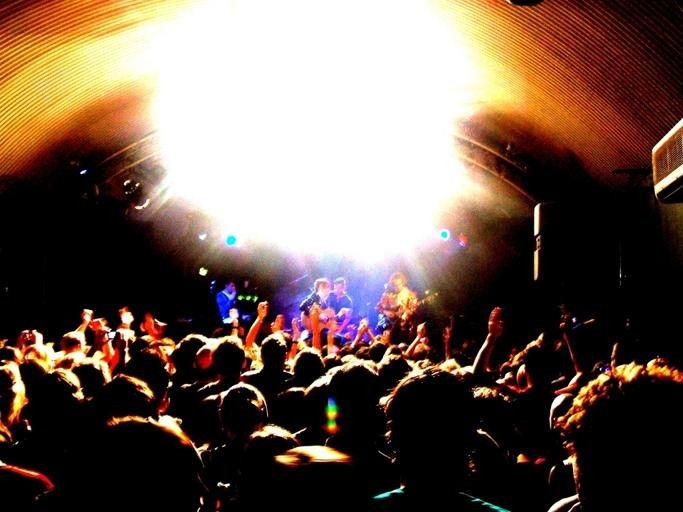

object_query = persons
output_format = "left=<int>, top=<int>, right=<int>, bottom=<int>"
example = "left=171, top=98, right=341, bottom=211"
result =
left=373, top=271, right=418, bottom=336
left=299, top=277, right=353, bottom=348
left=215, top=279, right=250, bottom=337
left=325, top=277, right=352, bottom=334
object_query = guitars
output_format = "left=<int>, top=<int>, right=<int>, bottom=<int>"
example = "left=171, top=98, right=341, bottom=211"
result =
left=301, top=309, right=367, bottom=334
left=377, top=293, right=440, bottom=330
